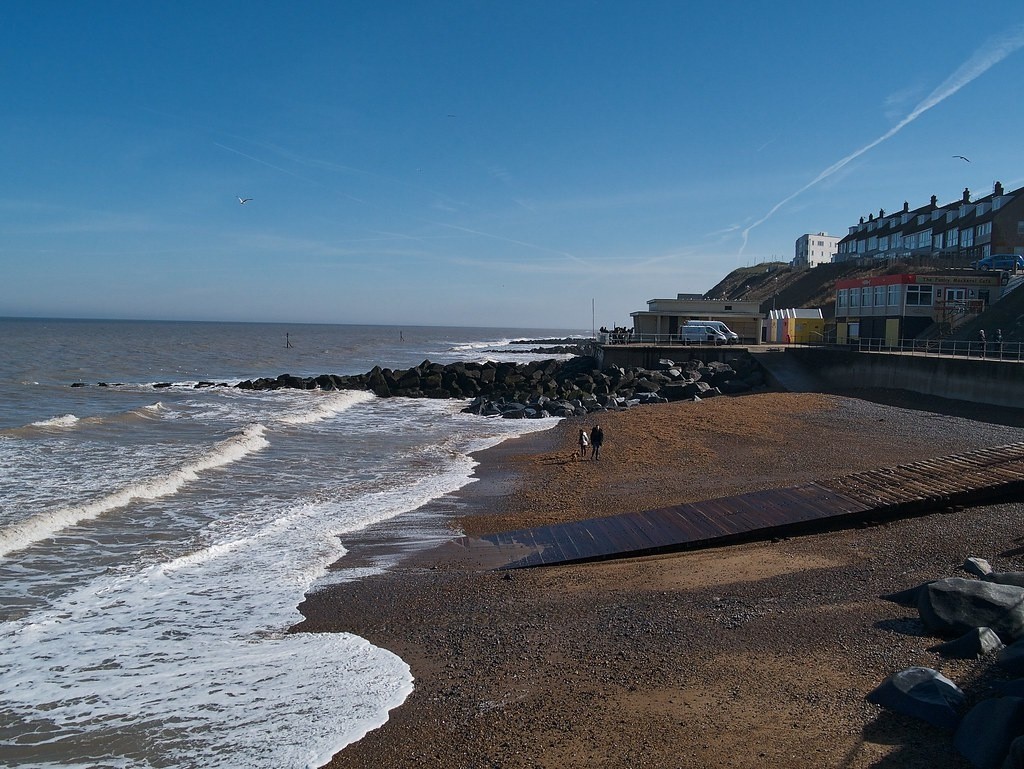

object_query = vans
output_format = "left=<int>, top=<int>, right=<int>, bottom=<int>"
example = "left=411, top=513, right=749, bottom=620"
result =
left=970, top=255, right=1024, bottom=271
left=687, top=321, right=738, bottom=344
left=682, top=326, right=727, bottom=346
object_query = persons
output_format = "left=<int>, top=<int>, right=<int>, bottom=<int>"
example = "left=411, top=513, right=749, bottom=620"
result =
left=994, top=328, right=1003, bottom=358
left=590, top=424, right=604, bottom=461
left=978, top=330, right=987, bottom=357
left=599, top=321, right=635, bottom=345
left=578, top=429, right=590, bottom=457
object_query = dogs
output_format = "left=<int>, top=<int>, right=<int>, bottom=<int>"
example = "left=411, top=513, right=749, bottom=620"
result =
left=571, top=450, right=579, bottom=463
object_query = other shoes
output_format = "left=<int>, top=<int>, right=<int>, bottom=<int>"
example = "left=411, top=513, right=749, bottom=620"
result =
left=590, top=457, right=592, bottom=460
left=595, top=459, right=600, bottom=461
